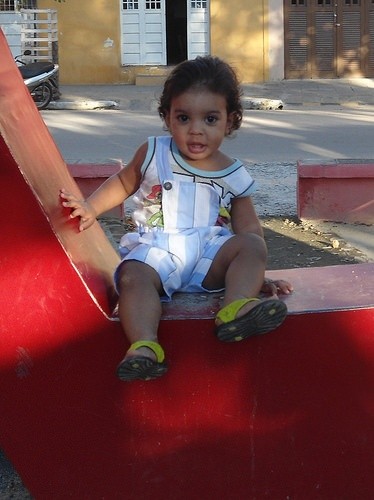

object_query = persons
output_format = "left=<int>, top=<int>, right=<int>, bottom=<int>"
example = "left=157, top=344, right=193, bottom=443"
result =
left=57, top=55, right=294, bottom=383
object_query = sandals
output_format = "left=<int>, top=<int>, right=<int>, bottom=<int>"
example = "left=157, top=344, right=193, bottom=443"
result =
left=215, top=296, right=287, bottom=343
left=116, top=339, right=168, bottom=381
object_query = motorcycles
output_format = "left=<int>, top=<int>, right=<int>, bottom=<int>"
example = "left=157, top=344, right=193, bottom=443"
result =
left=14, top=50, right=59, bottom=114
